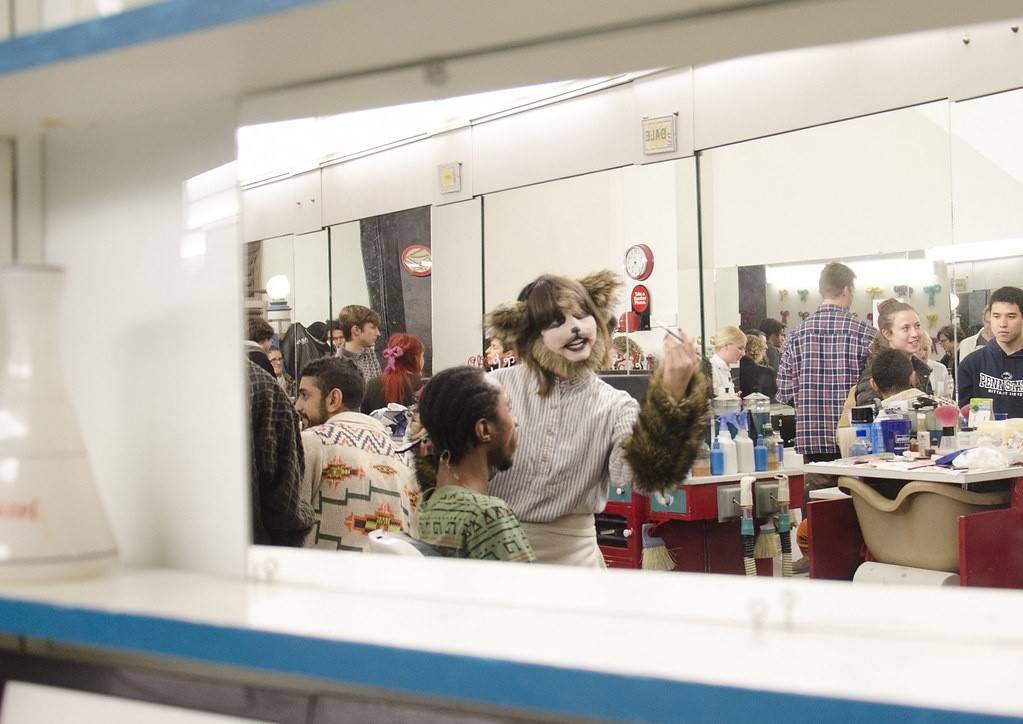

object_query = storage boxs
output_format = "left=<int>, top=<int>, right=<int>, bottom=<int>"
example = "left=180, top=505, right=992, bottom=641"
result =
left=838, top=477, right=1011, bottom=573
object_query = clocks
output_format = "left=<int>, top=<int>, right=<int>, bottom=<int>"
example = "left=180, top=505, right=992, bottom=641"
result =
left=624, top=244, right=653, bottom=281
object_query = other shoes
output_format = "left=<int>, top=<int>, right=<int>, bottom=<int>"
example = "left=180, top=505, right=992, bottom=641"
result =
left=792, top=557, right=809, bottom=572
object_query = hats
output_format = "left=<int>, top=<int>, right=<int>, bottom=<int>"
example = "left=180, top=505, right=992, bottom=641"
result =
left=303, top=322, right=330, bottom=351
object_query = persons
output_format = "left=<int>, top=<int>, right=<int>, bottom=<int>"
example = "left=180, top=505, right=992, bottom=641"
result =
left=855, top=298, right=967, bottom=455
left=958, top=286, right=1023, bottom=421
left=248, top=305, right=429, bottom=554
left=707, top=326, right=748, bottom=412
left=477, top=268, right=715, bottom=569
left=772, top=262, right=881, bottom=492
left=485, top=336, right=519, bottom=372
left=419, top=366, right=538, bottom=565
left=740, top=316, right=788, bottom=403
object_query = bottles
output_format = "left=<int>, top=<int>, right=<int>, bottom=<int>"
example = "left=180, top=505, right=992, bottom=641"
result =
left=849, top=431, right=874, bottom=456
left=709, top=417, right=783, bottom=476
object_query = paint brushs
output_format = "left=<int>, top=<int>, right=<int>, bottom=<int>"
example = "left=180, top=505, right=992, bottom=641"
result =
left=648, top=316, right=712, bottom=364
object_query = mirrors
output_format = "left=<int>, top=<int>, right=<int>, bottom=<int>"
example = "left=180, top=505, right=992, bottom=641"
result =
left=231, top=0, right=1023, bottom=594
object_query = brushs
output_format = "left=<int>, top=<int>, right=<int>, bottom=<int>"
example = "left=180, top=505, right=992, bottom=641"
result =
left=639, top=523, right=678, bottom=571
left=754, top=515, right=782, bottom=559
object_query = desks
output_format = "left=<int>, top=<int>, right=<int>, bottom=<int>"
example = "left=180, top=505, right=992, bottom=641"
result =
left=593, top=465, right=806, bottom=576
left=804, top=451, right=1023, bottom=491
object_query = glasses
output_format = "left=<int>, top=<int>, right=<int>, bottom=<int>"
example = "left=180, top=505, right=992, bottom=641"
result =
left=939, top=338, right=949, bottom=344
left=270, top=358, right=284, bottom=363
